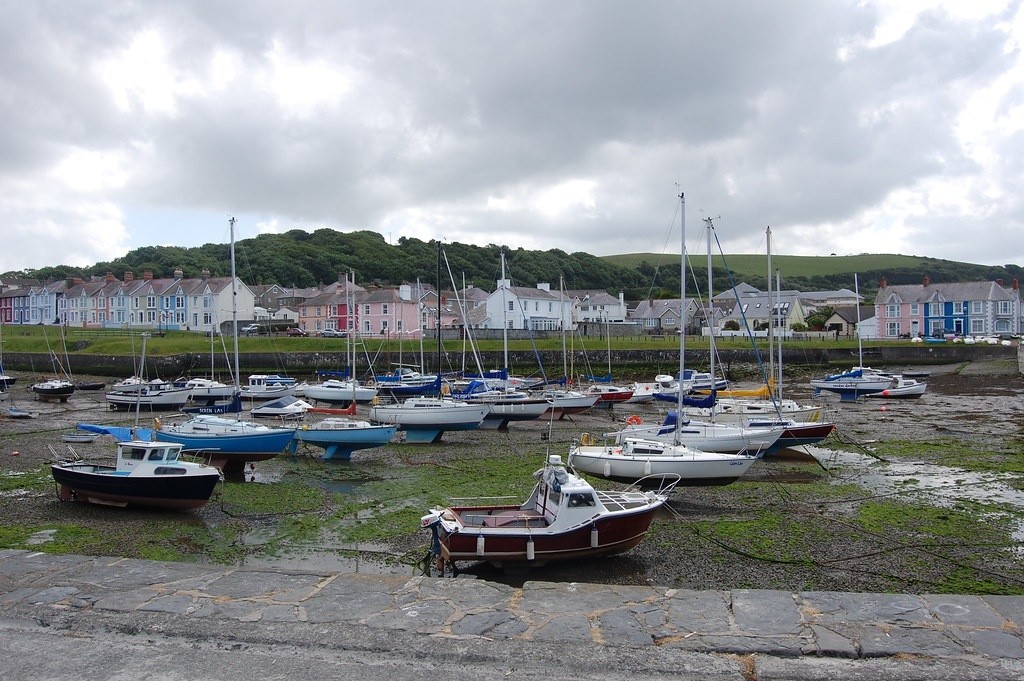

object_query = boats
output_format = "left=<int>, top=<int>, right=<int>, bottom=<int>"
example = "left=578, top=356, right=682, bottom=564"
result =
left=677, top=368, right=729, bottom=391
left=865, top=374, right=928, bottom=400
left=422, top=455, right=681, bottom=567
left=1, top=376, right=16, bottom=404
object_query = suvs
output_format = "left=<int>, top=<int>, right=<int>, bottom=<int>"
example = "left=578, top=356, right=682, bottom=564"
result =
left=241, top=324, right=261, bottom=333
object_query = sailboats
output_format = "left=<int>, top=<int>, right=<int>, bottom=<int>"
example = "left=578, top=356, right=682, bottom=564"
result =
left=603, top=215, right=787, bottom=456
left=925, top=290, right=946, bottom=344
left=568, top=191, right=770, bottom=483
left=104, top=214, right=684, bottom=467
left=51, top=331, right=219, bottom=512
left=811, top=272, right=895, bottom=391
left=30, top=319, right=75, bottom=404
left=681, top=267, right=835, bottom=450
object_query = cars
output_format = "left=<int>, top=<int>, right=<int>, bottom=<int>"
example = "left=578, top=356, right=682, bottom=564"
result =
left=320, top=328, right=347, bottom=338
left=932, top=329, right=963, bottom=339
left=285, top=329, right=309, bottom=336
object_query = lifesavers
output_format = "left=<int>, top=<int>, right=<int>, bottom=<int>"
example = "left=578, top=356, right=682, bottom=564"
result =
left=373, top=396, right=379, bottom=405
left=568, top=379, right=573, bottom=384
left=627, top=415, right=641, bottom=425
left=582, top=434, right=593, bottom=445
left=441, top=386, right=450, bottom=395
left=154, top=418, right=161, bottom=429
